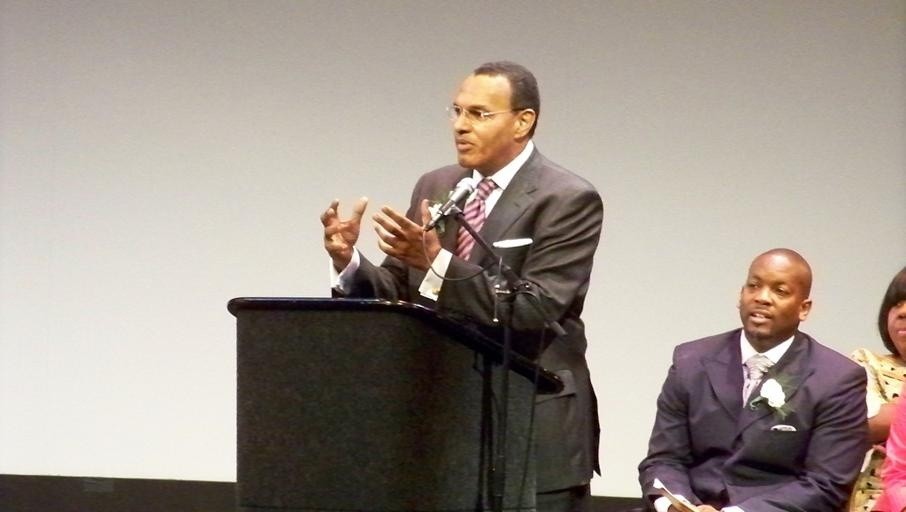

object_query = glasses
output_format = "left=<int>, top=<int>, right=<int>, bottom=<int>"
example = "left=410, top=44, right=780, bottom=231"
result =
left=447, top=106, right=520, bottom=125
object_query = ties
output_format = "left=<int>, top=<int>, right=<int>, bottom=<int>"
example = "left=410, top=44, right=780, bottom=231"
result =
left=457, top=178, right=497, bottom=263
left=743, top=356, right=773, bottom=406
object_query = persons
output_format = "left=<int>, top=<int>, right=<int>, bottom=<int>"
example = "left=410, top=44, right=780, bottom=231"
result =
left=847, top=266, right=906, bottom=512
left=637, top=245, right=869, bottom=511
left=317, top=60, right=603, bottom=511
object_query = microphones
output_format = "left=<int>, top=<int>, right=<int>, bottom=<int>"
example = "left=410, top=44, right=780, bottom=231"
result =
left=424, top=176, right=478, bottom=243
